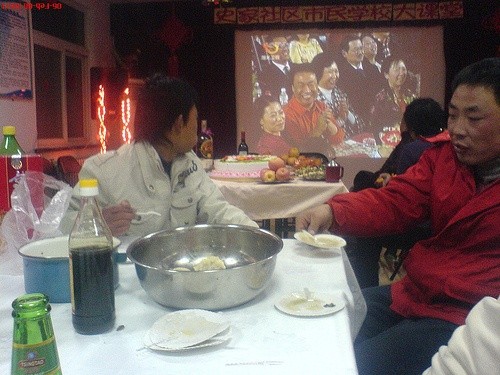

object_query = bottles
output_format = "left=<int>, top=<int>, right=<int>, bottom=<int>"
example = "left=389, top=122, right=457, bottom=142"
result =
left=0, top=125, right=26, bottom=155
left=252, top=81, right=262, bottom=103
left=9, top=292, right=62, bottom=375
left=326, top=156, right=344, bottom=183
left=238, top=130, right=248, bottom=155
left=279, top=87, right=289, bottom=106
left=196, top=119, right=213, bottom=159
left=68, top=179, right=116, bottom=336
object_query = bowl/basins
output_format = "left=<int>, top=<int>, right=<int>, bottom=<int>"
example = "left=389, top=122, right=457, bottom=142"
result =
left=125, top=223, right=284, bottom=309
left=18, top=235, right=123, bottom=303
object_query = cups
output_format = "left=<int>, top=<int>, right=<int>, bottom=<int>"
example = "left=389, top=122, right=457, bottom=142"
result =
left=362, top=138, right=376, bottom=156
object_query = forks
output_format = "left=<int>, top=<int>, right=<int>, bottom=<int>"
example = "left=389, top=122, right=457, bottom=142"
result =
left=302, top=229, right=326, bottom=245
left=304, top=287, right=320, bottom=311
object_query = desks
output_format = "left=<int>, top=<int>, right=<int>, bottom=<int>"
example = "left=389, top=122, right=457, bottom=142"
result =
left=0, top=237, right=368, bottom=375
left=211, top=177, right=350, bottom=237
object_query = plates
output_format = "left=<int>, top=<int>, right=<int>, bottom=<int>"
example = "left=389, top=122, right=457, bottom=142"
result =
left=304, top=177, right=325, bottom=181
left=294, top=232, right=347, bottom=249
left=256, top=179, right=290, bottom=184
left=143, top=308, right=233, bottom=352
left=275, top=293, right=345, bottom=317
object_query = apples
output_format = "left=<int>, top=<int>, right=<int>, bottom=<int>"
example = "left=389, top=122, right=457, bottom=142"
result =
left=260, top=158, right=290, bottom=182
left=281, top=147, right=305, bottom=165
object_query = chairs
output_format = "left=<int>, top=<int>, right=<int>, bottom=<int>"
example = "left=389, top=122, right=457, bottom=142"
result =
left=58, top=155, right=82, bottom=187
left=253, top=152, right=329, bottom=239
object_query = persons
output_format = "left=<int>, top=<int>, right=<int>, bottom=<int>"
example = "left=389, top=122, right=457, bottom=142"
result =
left=295, top=58, right=500, bottom=375
left=252, top=34, right=419, bottom=156
left=58, top=74, right=259, bottom=241
left=422, top=295, right=500, bottom=375
left=346, top=97, right=448, bottom=286
